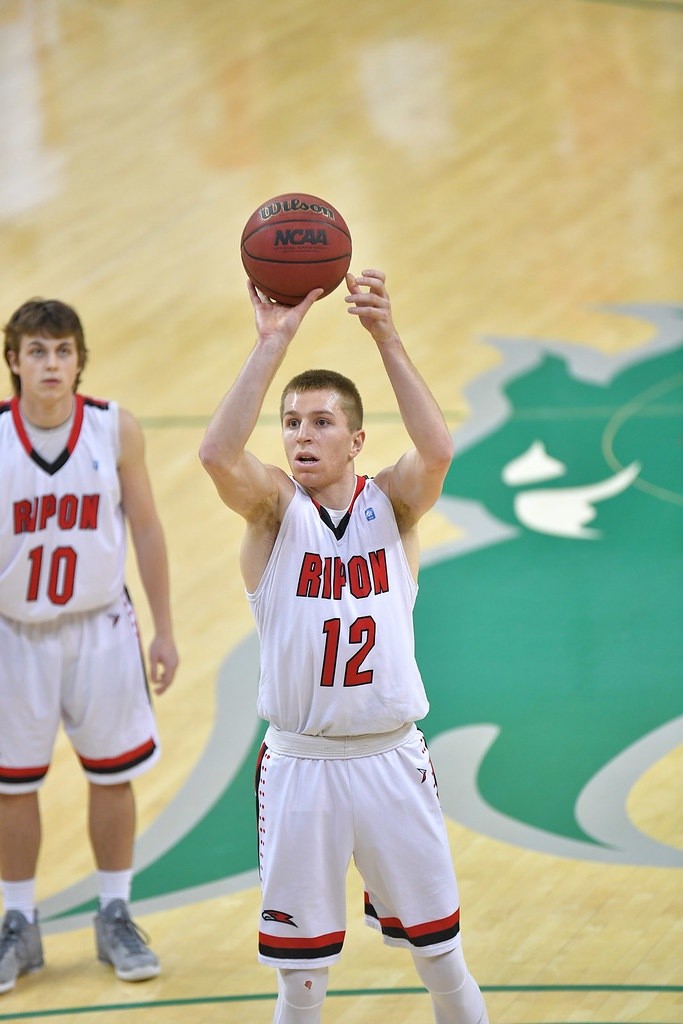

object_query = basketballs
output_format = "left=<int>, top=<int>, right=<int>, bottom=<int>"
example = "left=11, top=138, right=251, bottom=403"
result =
left=239, top=192, right=356, bottom=308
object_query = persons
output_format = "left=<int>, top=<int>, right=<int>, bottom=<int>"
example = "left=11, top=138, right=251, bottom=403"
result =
left=199, top=271, right=490, bottom=1024
left=1, top=297, right=179, bottom=992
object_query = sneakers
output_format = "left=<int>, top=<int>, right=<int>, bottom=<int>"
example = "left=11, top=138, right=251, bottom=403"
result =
left=0, top=909, right=44, bottom=994
left=94, top=899, right=161, bottom=982
